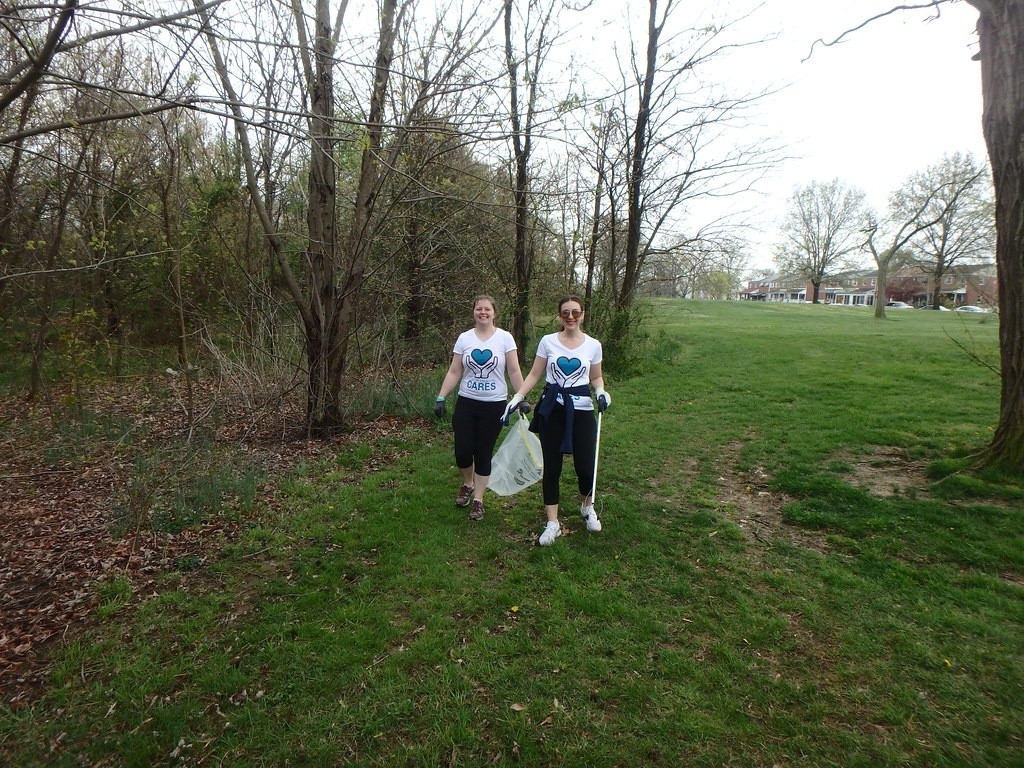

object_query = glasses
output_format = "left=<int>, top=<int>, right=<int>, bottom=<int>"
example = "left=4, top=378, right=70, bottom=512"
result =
left=558, top=311, right=584, bottom=319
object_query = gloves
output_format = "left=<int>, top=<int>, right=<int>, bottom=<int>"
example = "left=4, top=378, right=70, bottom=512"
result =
left=519, top=401, right=530, bottom=416
left=500, top=394, right=525, bottom=426
left=435, top=397, right=445, bottom=418
left=595, top=388, right=611, bottom=410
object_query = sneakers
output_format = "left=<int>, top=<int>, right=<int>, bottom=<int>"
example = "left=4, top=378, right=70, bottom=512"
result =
left=468, top=498, right=484, bottom=520
left=455, top=482, right=475, bottom=506
left=580, top=502, right=601, bottom=532
left=539, top=521, right=562, bottom=545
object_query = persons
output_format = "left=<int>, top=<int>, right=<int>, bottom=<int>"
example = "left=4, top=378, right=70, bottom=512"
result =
left=434, top=295, right=531, bottom=521
left=501, top=297, right=611, bottom=547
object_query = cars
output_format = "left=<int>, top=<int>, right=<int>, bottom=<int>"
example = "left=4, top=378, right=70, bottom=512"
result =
left=955, top=306, right=985, bottom=312
left=885, top=301, right=914, bottom=309
left=918, top=305, right=951, bottom=311
